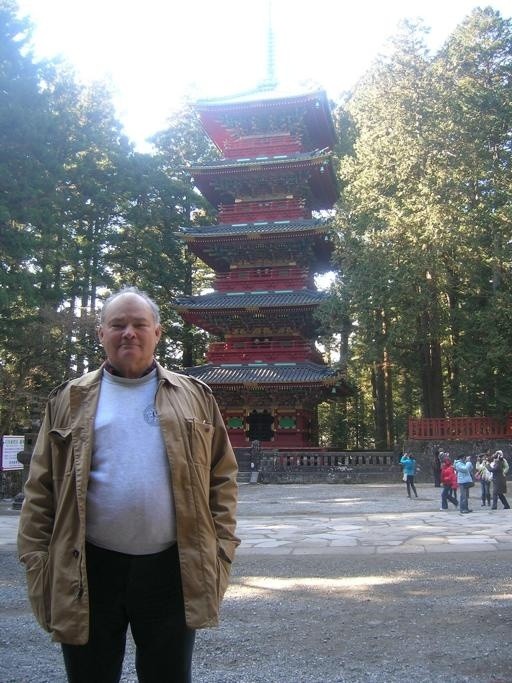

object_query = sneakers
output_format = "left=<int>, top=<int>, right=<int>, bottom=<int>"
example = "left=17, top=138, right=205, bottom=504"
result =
left=406, top=494, right=511, bottom=516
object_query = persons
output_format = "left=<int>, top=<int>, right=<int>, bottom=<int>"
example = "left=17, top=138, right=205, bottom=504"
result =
left=399, top=442, right=510, bottom=514
left=16, top=288, right=240, bottom=683
